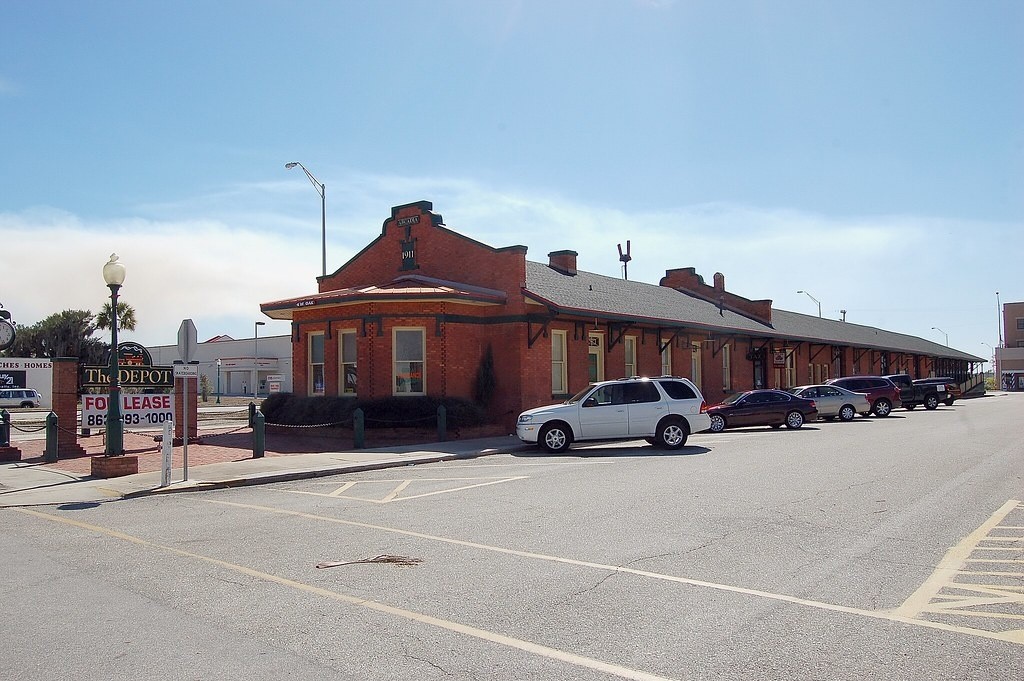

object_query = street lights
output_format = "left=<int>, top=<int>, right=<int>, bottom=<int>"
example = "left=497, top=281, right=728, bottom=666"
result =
left=283, top=161, right=327, bottom=279
left=254, top=321, right=266, bottom=399
left=215, top=358, right=222, bottom=404
left=996, top=290, right=1002, bottom=346
left=102, top=252, right=126, bottom=458
left=932, top=327, right=949, bottom=348
left=796, top=290, right=822, bottom=319
left=980, top=341, right=995, bottom=385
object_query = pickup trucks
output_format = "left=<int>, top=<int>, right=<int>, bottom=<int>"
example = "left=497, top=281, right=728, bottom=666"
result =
left=880, top=373, right=948, bottom=410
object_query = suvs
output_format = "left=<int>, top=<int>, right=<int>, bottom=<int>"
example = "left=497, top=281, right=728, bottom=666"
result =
left=911, top=376, right=961, bottom=409
left=515, top=375, right=712, bottom=455
left=822, top=375, right=902, bottom=418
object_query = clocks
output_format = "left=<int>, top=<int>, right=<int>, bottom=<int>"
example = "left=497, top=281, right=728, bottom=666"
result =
left=0, top=317, right=16, bottom=351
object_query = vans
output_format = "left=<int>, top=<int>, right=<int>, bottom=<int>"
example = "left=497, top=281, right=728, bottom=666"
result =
left=1, top=388, right=42, bottom=409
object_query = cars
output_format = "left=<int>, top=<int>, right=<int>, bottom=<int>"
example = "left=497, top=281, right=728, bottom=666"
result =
left=786, top=384, right=872, bottom=422
left=701, top=387, right=818, bottom=434
